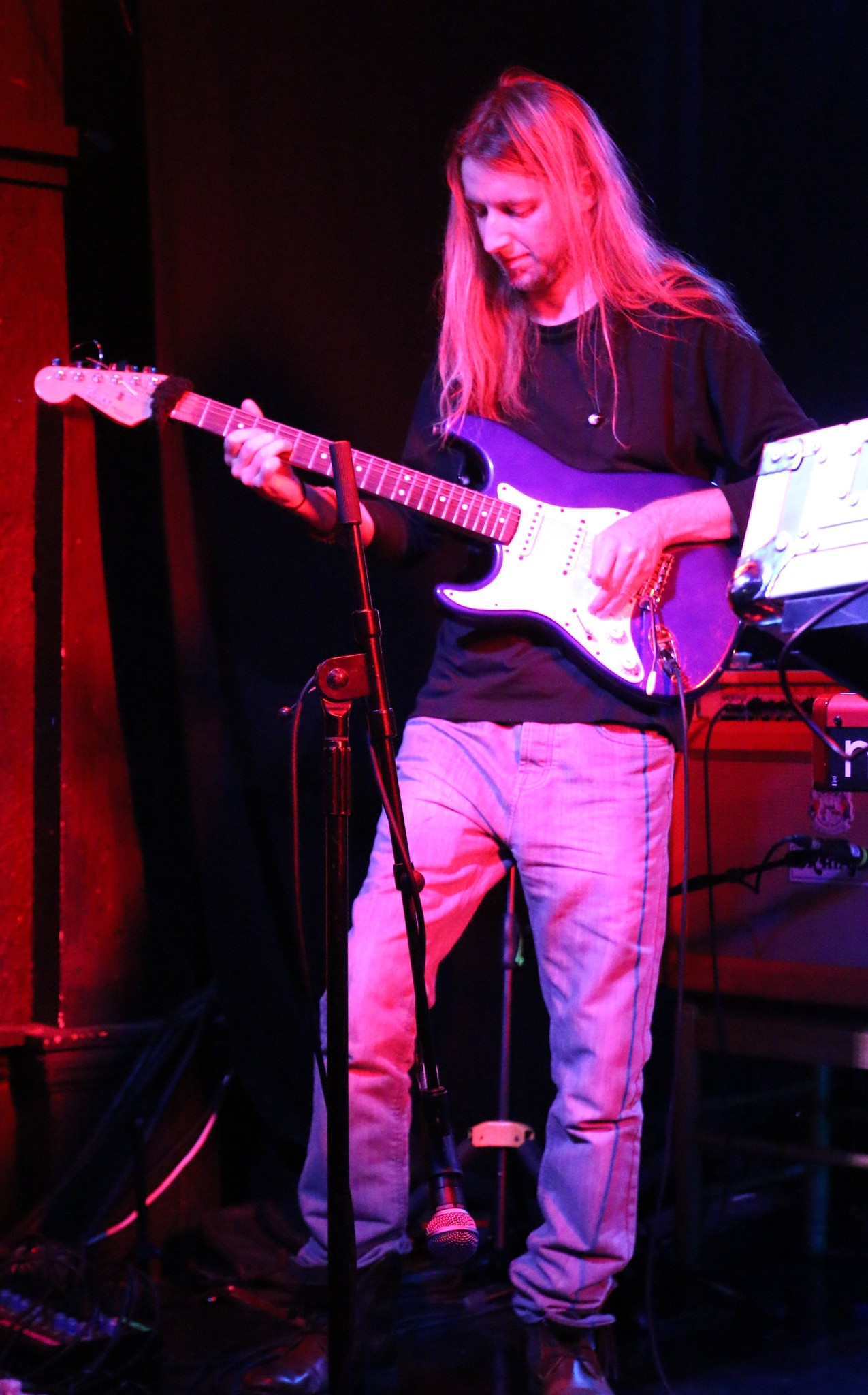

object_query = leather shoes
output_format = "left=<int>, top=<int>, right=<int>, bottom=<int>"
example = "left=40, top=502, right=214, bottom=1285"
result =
left=526, top=1326, right=611, bottom=1395
left=230, top=1333, right=327, bottom=1395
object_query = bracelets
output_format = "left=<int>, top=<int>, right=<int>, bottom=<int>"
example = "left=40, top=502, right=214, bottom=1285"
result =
left=291, top=478, right=307, bottom=510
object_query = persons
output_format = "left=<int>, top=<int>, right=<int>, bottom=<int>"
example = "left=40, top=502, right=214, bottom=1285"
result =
left=222, top=67, right=820, bottom=1395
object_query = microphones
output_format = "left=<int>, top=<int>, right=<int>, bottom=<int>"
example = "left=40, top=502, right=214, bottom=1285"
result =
left=412, top=1058, right=481, bottom=1265
left=791, top=834, right=867, bottom=869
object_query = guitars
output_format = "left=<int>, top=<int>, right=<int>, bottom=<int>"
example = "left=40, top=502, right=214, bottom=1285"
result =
left=35, top=347, right=747, bottom=711
